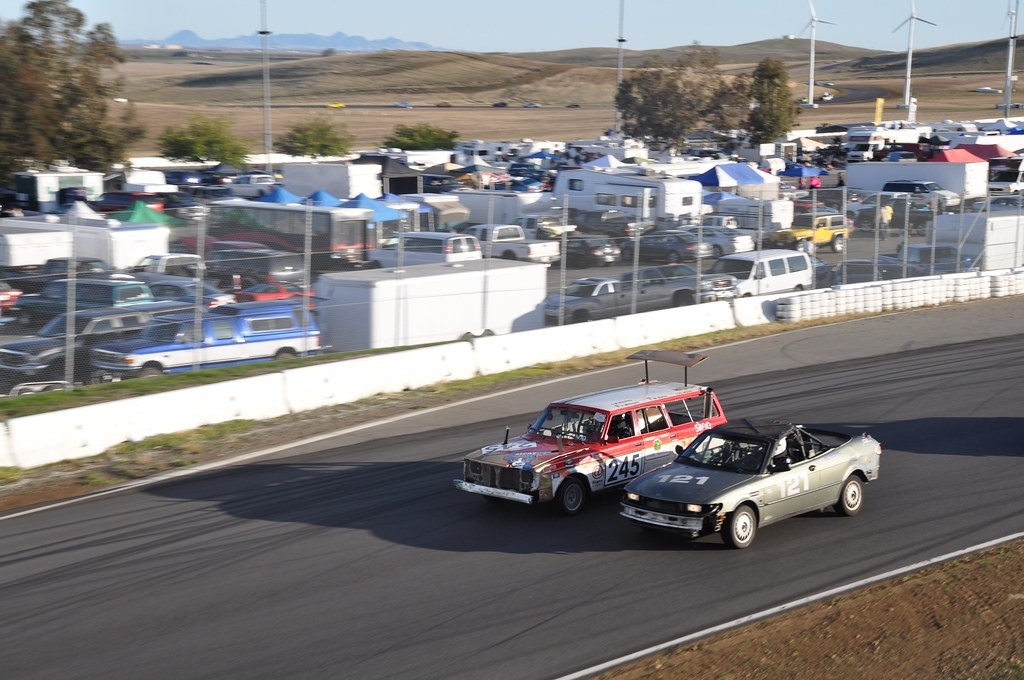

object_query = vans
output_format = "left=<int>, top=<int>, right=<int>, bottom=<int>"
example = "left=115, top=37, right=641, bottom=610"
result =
left=701, top=247, right=814, bottom=299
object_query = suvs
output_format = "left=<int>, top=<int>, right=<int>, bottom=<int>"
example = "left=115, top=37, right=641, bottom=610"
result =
left=883, top=180, right=961, bottom=211
left=769, top=211, right=850, bottom=254
left=0, top=300, right=207, bottom=387
left=86, top=299, right=324, bottom=380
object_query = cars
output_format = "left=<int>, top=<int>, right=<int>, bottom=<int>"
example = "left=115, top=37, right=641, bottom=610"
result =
left=0, top=117, right=1024, bottom=330
left=619, top=417, right=882, bottom=549
left=453, top=350, right=728, bottom=517
left=545, top=275, right=622, bottom=323
left=836, top=260, right=885, bottom=286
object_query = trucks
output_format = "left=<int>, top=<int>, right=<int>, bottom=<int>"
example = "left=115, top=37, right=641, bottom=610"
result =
left=846, top=125, right=921, bottom=163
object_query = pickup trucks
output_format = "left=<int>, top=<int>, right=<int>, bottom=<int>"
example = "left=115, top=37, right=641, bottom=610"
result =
left=873, top=243, right=978, bottom=279
left=461, top=224, right=561, bottom=267
left=558, top=262, right=742, bottom=325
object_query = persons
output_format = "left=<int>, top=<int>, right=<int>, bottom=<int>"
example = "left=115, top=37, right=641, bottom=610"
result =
left=879, top=202, right=895, bottom=241
left=797, top=237, right=819, bottom=269
left=586, top=414, right=633, bottom=445
left=739, top=436, right=793, bottom=471
left=798, top=171, right=847, bottom=193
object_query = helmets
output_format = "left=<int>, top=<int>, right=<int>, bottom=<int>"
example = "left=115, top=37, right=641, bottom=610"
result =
left=771, top=436, right=787, bottom=458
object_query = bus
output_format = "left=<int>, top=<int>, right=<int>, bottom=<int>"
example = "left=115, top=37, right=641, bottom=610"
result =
left=204, top=197, right=374, bottom=267
